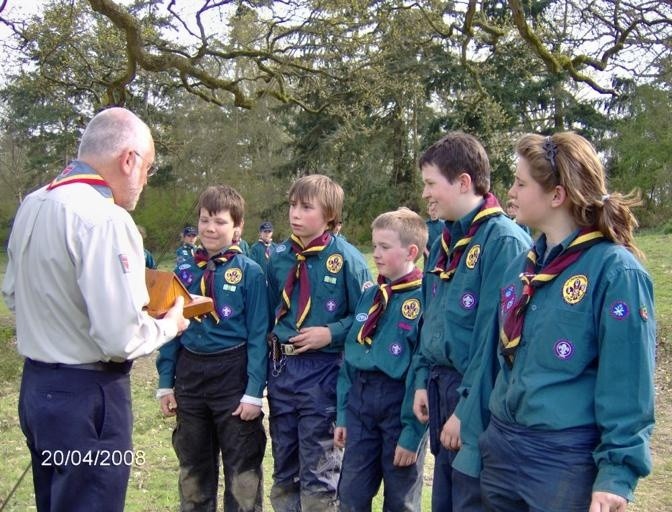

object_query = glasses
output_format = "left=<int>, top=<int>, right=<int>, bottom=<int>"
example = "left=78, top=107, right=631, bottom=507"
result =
left=135, top=152, right=158, bottom=177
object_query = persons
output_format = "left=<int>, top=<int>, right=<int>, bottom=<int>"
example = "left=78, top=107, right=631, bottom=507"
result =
left=425, top=200, right=531, bottom=250
left=413, top=132, right=534, bottom=512
left=136, top=223, right=346, bottom=272
left=155, top=186, right=269, bottom=512
left=2, top=106, right=191, bottom=511
left=267, top=173, right=375, bottom=512
left=451, top=131, right=655, bottom=512
left=334, top=207, right=430, bottom=512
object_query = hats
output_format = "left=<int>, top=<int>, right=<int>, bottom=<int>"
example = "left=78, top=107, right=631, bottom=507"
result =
left=184, top=227, right=197, bottom=236
left=260, top=222, right=273, bottom=231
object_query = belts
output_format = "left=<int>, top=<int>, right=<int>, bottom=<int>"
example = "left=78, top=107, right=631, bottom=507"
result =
left=278, top=344, right=314, bottom=356
